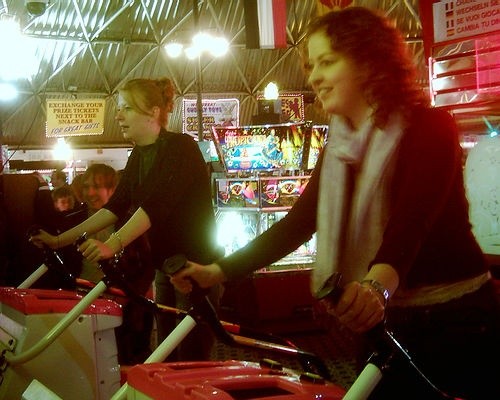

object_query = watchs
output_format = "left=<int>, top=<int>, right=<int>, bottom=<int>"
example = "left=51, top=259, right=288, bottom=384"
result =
left=361, top=280, right=390, bottom=304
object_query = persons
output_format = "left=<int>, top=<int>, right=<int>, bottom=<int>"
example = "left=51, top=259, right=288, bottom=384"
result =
left=167, top=7, right=499, bottom=400
left=34, top=169, right=123, bottom=210
left=58, top=164, right=120, bottom=285
left=28, top=78, right=219, bottom=365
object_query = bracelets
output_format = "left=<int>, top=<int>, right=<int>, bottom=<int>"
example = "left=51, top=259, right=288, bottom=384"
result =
left=110, top=232, right=124, bottom=264
